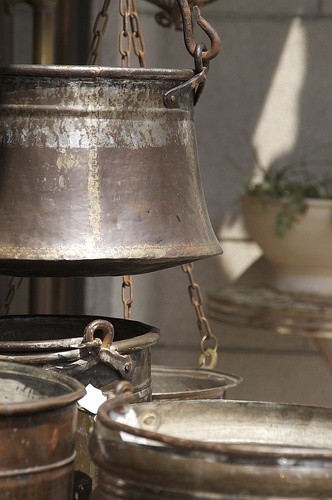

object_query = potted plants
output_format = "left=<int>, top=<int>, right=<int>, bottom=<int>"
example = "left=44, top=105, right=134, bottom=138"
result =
left=234, top=130, right=332, bottom=293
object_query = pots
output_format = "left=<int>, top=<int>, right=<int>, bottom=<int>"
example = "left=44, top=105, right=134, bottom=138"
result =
left=121, top=261, right=242, bottom=399
left=89, top=381, right=332, bottom=500
left=0, top=360, right=87, bottom=500
left=0, top=0, right=224, bottom=278
left=0, top=314, right=161, bottom=402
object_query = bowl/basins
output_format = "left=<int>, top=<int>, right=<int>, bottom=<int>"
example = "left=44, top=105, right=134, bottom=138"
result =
left=237, top=196, right=331, bottom=298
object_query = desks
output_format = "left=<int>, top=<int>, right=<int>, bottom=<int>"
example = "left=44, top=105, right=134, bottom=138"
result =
left=206, top=288, right=332, bottom=368
